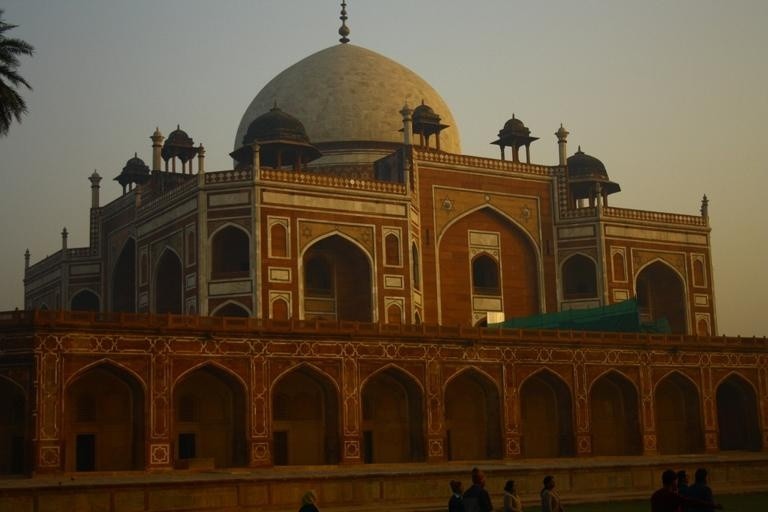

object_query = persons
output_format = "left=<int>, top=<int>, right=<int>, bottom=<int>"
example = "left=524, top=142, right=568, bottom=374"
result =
left=448, top=480, right=465, bottom=512
left=298, top=489, right=320, bottom=512
left=651, top=469, right=690, bottom=512
left=463, top=469, right=493, bottom=512
left=504, top=480, right=523, bottom=512
left=688, top=468, right=712, bottom=511
left=677, top=470, right=691, bottom=496
left=541, top=476, right=565, bottom=512
